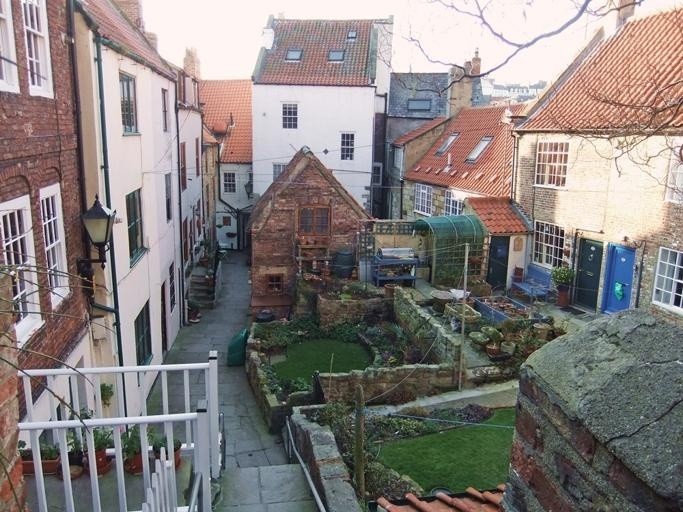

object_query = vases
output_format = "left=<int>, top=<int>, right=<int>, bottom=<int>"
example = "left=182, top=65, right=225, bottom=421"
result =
left=486, top=344, right=499, bottom=353
left=500, top=341, right=516, bottom=354
left=384, top=284, right=396, bottom=296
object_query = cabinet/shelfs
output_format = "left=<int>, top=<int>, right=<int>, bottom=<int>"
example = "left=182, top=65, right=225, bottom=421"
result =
left=372, top=255, right=417, bottom=288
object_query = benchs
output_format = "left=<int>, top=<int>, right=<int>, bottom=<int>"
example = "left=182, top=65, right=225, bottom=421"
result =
left=511, top=265, right=550, bottom=305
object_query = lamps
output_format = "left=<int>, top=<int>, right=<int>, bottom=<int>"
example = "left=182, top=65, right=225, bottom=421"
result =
left=77, top=194, right=116, bottom=270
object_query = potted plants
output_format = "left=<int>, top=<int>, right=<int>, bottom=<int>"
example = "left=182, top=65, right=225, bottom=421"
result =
left=154, top=437, right=182, bottom=466
left=81, top=430, right=112, bottom=468
left=550, top=267, right=575, bottom=305
left=120, top=423, right=154, bottom=468
left=17, top=440, right=60, bottom=474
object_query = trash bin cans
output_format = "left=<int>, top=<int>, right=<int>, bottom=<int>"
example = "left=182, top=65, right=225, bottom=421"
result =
left=188, top=295, right=201, bottom=323
left=227, top=328, right=248, bottom=367
